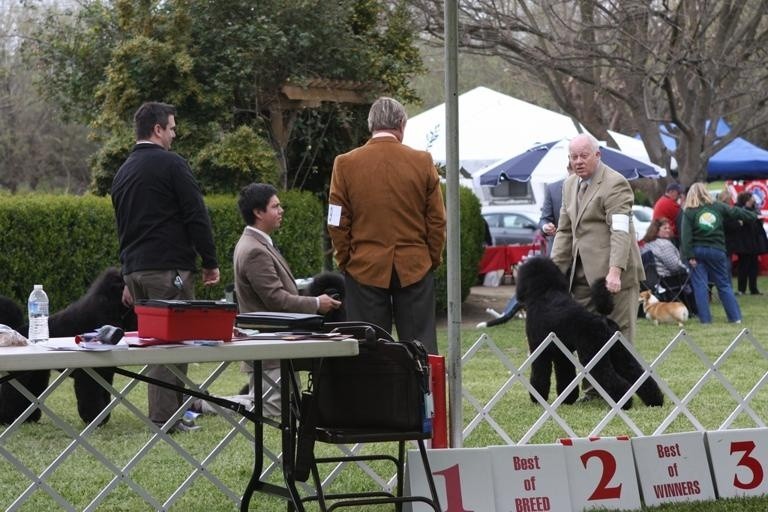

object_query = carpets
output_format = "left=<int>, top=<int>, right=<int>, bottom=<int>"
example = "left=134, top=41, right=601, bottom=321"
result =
left=577, top=182, right=588, bottom=213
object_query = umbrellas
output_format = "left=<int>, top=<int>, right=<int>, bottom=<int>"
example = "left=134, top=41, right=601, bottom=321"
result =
left=150, top=419, right=203, bottom=435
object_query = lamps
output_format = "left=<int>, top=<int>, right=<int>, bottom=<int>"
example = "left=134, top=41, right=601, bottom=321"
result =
left=287, top=321, right=443, bottom=511
left=639, top=249, right=695, bottom=318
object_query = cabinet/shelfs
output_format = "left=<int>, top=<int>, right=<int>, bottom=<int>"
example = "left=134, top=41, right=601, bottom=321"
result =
left=28, top=284, right=50, bottom=345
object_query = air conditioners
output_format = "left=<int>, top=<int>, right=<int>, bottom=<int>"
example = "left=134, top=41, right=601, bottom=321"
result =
left=0, top=268, right=137, bottom=427
left=639, top=290, right=689, bottom=327
left=515, top=254, right=664, bottom=410
left=303, top=270, right=346, bottom=323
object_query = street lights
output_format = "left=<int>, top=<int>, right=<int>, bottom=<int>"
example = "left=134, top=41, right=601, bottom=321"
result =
left=312, top=321, right=428, bottom=434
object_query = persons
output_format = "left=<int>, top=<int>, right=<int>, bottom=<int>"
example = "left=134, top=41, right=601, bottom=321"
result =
left=108, top=102, right=222, bottom=436
left=175, top=180, right=343, bottom=421
left=638, top=180, right=767, bottom=324
left=324, top=94, right=448, bottom=395
left=539, top=160, right=576, bottom=260
left=546, top=132, right=649, bottom=402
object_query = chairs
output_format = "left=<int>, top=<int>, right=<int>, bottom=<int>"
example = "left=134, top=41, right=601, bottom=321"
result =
left=575, top=391, right=604, bottom=403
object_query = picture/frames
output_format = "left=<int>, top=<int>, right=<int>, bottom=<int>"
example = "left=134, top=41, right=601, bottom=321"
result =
left=479, top=244, right=542, bottom=285
left=0, top=327, right=361, bottom=511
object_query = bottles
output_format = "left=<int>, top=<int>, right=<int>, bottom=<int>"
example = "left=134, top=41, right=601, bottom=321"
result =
left=666, top=183, right=685, bottom=193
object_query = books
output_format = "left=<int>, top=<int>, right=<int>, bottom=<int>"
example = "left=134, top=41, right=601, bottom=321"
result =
left=133, top=299, right=238, bottom=343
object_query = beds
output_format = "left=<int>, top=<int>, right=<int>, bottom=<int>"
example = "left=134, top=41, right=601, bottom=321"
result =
left=475, top=136, right=662, bottom=188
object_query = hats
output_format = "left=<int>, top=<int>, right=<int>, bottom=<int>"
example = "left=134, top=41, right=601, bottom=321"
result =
left=482, top=205, right=543, bottom=248
left=631, top=204, right=657, bottom=240
